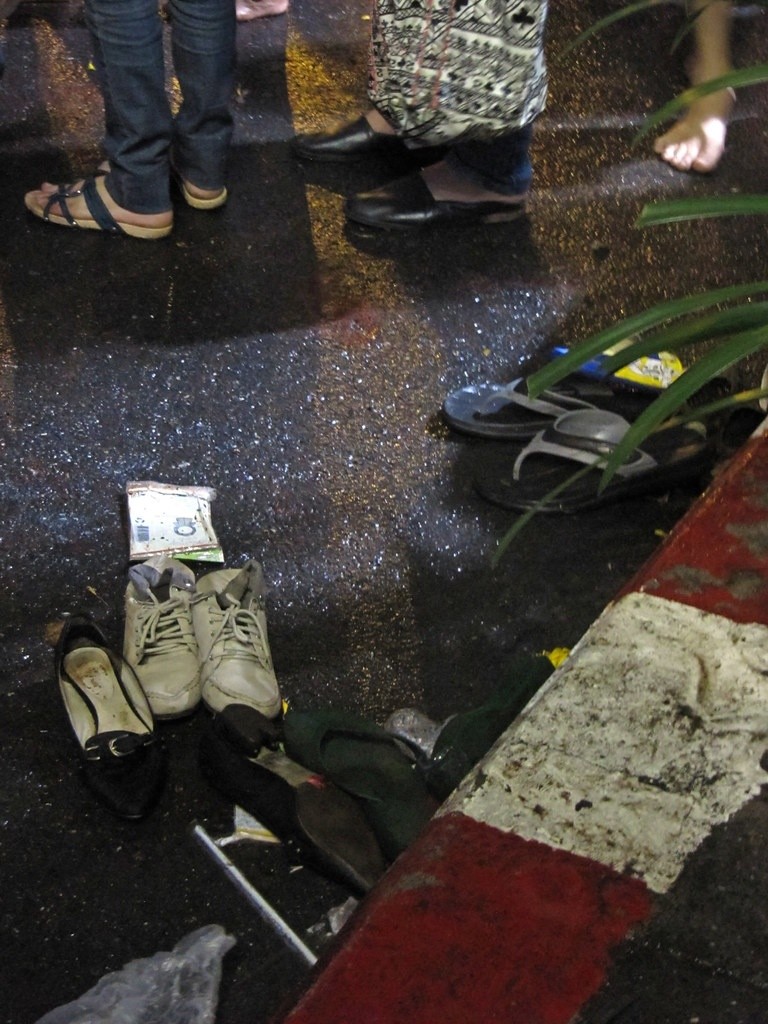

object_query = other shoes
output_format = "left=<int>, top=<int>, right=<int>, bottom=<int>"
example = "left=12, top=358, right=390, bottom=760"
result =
left=53, top=615, right=166, bottom=819
left=535, top=337, right=694, bottom=397
left=386, top=710, right=492, bottom=792
left=275, top=705, right=440, bottom=845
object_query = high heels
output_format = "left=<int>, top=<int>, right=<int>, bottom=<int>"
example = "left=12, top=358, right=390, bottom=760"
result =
left=199, top=704, right=387, bottom=895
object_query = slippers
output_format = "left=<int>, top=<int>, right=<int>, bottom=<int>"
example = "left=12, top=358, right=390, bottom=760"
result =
left=24, top=173, right=174, bottom=239
left=441, top=339, right=661, bottom=439
left=481, top=407, right=713, bottom=514
left=165, top=145, right=227, bottom=210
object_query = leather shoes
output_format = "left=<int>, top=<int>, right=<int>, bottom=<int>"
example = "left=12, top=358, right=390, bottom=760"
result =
left=342, top=167, right=526, bottom=234
left=288, top=116, right=472, bottom=163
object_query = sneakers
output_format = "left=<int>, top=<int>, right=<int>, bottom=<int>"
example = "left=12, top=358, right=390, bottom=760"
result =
left=187, top=555, right=283, bottom=720
left=124, top=555, right=201, bottom=722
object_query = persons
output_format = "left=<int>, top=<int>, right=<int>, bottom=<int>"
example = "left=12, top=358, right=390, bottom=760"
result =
left=25, top=0, right=236, bottom=239
left=291, top=0, right=549, bottom=230
left=236, top=0, right=288, bottom=21
left=653, top=0, right=737, bottom=172
